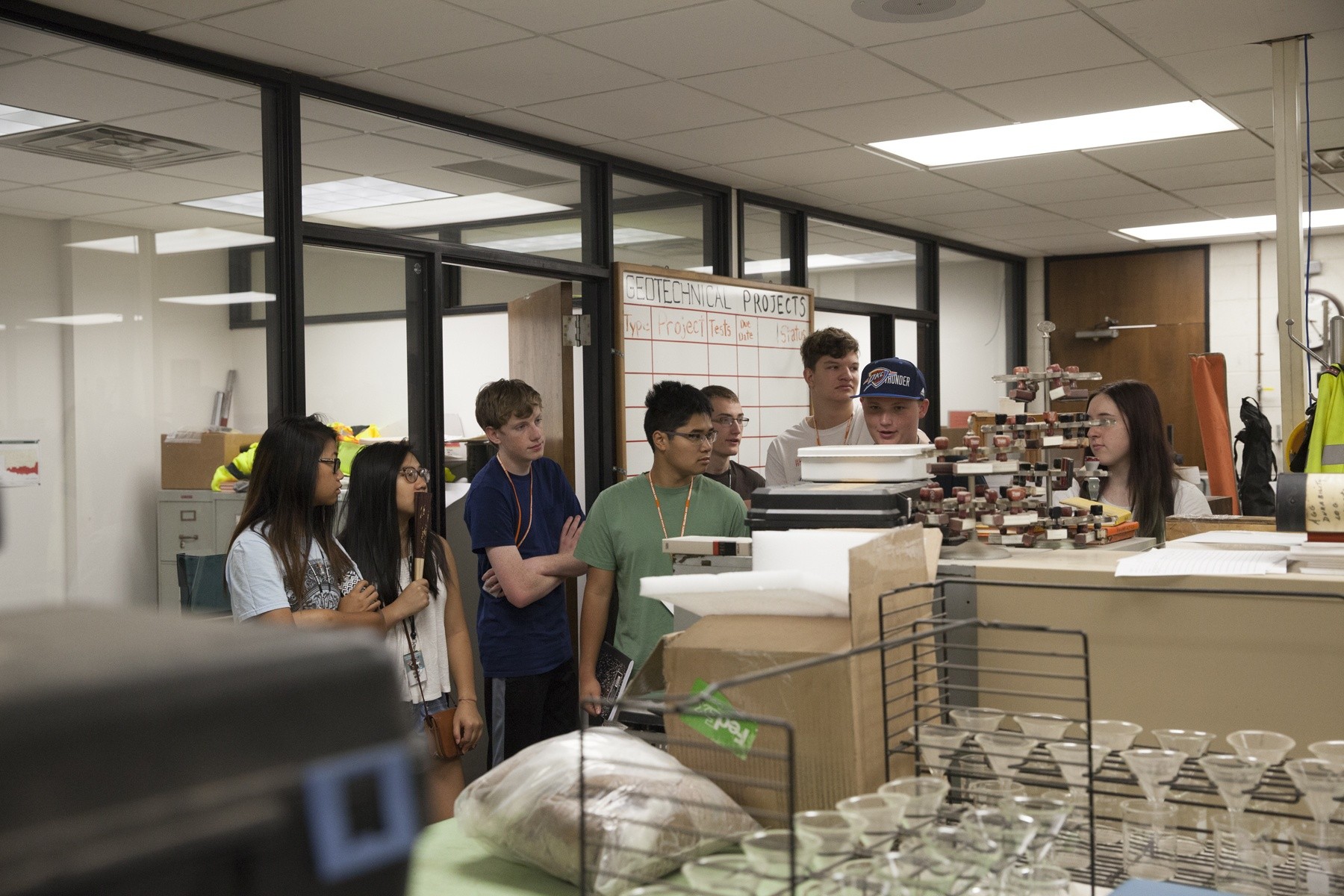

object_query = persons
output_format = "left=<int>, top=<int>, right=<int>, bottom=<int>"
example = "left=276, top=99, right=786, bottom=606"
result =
left=461, top=380, right=593, bottom=772
left=1055, top=379, right=1214, bottom=519
left=846, top=355, right=932, bottom=446
left=223, top=415, right=388, bottom=641
left=330, top=439, right=486, bottom=833
left=766, top=325, right=876, bottom=502
left=693, top=384, right=767, bottom=514
left=572, top=381, right=752, bottom=732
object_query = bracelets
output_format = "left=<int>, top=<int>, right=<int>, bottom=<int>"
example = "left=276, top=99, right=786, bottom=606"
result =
left=457, top=696, right=478, bottom=706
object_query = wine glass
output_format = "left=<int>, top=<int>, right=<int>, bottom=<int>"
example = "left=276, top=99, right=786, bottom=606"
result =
left=631, top=778, right=1078, bottom=895
left=911, top=690, right=1344, bottom=828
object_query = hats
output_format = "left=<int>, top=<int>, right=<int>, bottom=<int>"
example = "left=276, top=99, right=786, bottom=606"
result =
left=851, top=358, right=926, bottom=405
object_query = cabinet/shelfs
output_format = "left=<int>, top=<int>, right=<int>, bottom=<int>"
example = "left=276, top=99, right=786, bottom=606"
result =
left=877, top=574, right=1344, bottom=895
left=578, top=616, right=1100, bottom=895
left=155, top=491, right=246, bottom=616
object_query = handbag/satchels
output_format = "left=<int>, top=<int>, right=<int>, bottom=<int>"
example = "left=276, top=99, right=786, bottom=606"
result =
left=387, top=539, right=466, bottom=760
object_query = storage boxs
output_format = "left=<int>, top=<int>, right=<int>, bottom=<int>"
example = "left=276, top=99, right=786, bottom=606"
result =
left=660, top=521, right=943, bottom=826
left=160, top=433, right=264, bottom=489
left=799, top=445, right=939, bottom=483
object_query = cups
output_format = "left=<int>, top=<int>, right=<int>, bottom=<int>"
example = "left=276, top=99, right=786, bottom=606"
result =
left=1119, top=803, right=1178, bottom=884
left=1212, top=810, right=1278, bottom=896
left=1288, top=821, right=1344, bottom=896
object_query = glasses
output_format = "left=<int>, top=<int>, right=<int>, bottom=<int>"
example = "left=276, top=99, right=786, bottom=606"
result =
left=662, top=430, right=718, bottom=445
left=398, top=467, right=431, bottom=482
left=712, top=416, right=749, bottom=428
left=317, top=457, right=341, bottom=473
